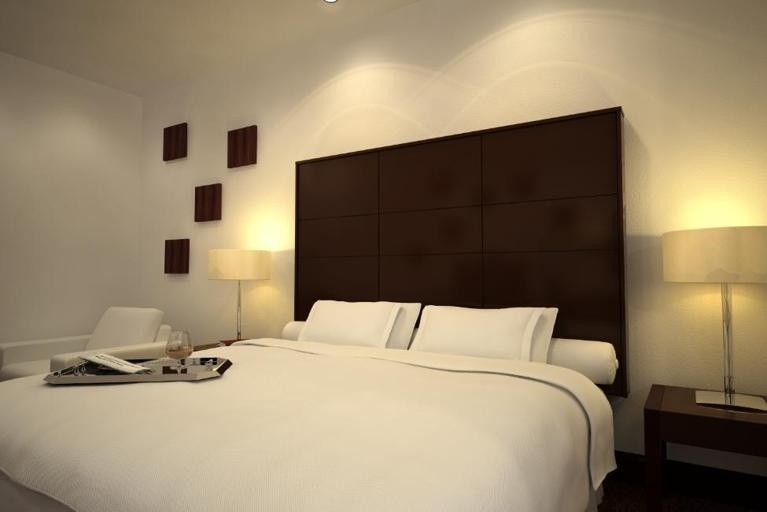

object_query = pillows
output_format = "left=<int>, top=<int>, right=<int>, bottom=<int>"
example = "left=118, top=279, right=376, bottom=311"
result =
left=297, top=300, right=402, bottom=349
left=531, top=308, right=559, bottom=364
left=409, top=304, right=541, bottom=363
left=385, top=300, right=421, bottom=349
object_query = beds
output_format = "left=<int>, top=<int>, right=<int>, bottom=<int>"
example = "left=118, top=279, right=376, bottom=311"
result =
left=0, top=98, right=631, bottom=511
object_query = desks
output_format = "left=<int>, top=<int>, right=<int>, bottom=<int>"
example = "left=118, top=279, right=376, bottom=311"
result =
left=642, top=383, right=767, bottom=510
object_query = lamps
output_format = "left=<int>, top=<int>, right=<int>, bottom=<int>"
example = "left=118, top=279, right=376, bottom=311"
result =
left=206, top=248, right=273, bottom=345
left=660, top=224, right=767, bottom=415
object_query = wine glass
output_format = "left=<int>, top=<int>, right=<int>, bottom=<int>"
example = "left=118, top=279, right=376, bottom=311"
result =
left=164, top=328, right=192, bottom=372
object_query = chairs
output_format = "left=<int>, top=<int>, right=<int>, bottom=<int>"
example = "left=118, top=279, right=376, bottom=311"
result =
left=3, top=307, right=193, bottom=380
